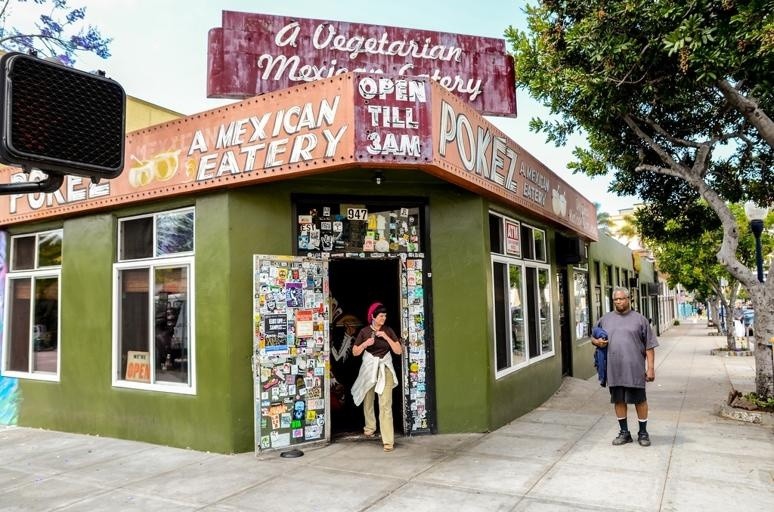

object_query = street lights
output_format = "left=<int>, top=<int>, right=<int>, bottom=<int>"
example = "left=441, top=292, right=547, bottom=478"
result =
left=744, top=197, right=770, bottom=283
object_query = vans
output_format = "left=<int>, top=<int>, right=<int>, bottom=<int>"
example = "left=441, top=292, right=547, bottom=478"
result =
left=155, top=293, right=187, bottom=367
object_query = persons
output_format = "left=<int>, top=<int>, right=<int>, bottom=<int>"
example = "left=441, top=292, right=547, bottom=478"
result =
left=284, top=365, right=289, bottom=373
left=318, top=337, right=322, bottom=344
left=259, top=284, right=268, bottom=292
left=590, top=287, right=658, bottom=446
left=697, top=308, right=702, bottom=318
left=329, top=323, right=357, bottom=407
left=351, top=302, right=402, bottom=452
left=290, top=325, right=294, bottom=332
left=290, top=270, right=299, bottom=280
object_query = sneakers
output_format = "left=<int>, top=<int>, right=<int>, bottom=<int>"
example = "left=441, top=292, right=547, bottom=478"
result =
left=384, top=444, right=393, bottom=452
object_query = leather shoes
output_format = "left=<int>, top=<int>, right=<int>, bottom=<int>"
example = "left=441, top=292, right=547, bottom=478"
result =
left=612, top=431, right=632, bottom=445
left=638, top=431, right=650, bottom=446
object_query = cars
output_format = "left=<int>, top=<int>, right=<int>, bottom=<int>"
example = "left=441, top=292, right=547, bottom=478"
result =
left=34, top=324, right=53, bottom=348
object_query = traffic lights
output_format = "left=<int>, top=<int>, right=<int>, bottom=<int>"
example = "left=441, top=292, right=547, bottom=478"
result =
left=0, top=48, right=127, bottom=183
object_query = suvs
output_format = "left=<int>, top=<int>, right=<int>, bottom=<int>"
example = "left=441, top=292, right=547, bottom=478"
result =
left=509, top=301, right=549, bottom=349
